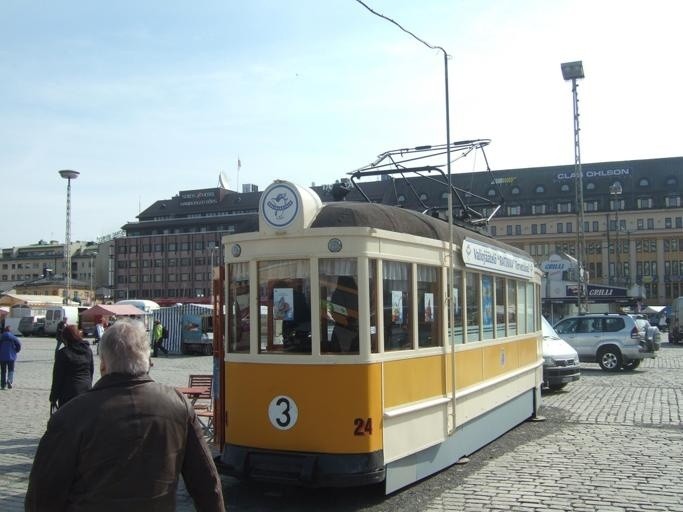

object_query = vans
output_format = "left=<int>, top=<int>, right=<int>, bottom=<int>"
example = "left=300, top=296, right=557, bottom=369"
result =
left=485, top=304, right=582, bottom=391
left=0, top=299, right=162, bottom=338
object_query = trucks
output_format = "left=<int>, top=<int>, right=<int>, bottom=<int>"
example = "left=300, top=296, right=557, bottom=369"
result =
left=665, top=297, right=682, bottom=345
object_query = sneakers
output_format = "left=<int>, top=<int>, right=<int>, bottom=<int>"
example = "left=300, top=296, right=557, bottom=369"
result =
left=7, top=379, right=12, bottom=389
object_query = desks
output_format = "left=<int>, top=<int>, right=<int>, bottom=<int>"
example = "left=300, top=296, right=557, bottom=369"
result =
left=174, top=386, right=208, bottom=407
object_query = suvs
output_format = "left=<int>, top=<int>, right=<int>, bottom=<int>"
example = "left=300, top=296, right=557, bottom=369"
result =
left=552, top=311, right=660, bottom=374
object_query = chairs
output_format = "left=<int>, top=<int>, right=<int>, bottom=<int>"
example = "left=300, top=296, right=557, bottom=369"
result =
left=185, top=374, right=214, bottom=437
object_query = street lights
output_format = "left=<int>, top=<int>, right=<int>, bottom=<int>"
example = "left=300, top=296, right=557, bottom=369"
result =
left=559, top=59, right=589, bottom=316
left=58, top=169, right=80, bottom=303
left=608, top=181, right=623, bottom=285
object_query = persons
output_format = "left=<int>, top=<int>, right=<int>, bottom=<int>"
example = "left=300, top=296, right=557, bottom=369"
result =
left=94, top=318, right=104, bottom=356
left=0, top=325, right=20, bottom=389
left=47, top=324, right=93, bottom=408
left=22, top=316, right=227, bottom=511
left=152, top=320, right=169, bottom=356
left=51, top=318, right=73, bottom=356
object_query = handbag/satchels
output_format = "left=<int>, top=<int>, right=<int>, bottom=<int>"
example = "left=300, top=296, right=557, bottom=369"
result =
left=162, top=327, right=169, bottom=338
